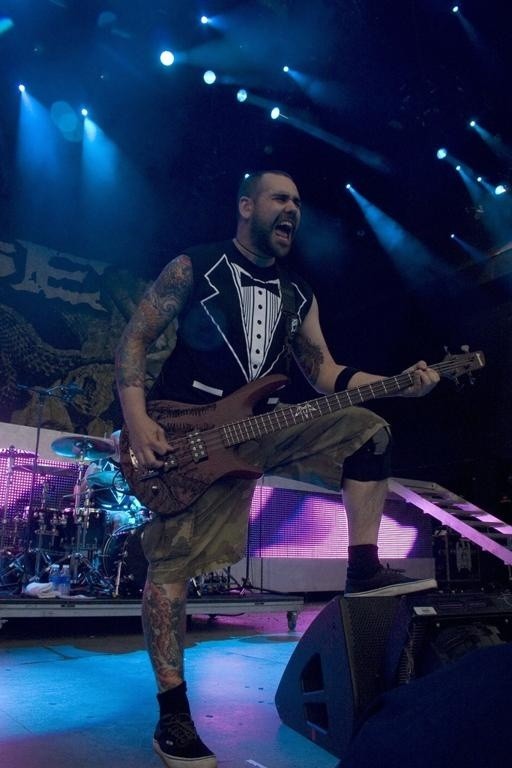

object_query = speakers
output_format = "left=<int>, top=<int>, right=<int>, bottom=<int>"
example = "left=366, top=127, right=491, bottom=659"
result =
left=434, top=513, right=512, bottom=591
left=275, top=579, right=441, bottom=768
left=374, top=590, right=512, bottom=691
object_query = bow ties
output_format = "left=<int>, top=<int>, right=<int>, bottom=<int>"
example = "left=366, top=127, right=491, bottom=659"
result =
left=239, top=270, right=280, bottom=298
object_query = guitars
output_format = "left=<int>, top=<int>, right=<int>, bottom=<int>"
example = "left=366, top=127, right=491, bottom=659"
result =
left=119, top=346, right=487, bottom=518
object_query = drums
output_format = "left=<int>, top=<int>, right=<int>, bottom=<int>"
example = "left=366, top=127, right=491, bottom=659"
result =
left=18, top=505, right=35, bottom=553
left=27, top=507, right=71, bottom=558
left=57, top=507, right=110, bottom=551
left=98, top=523, right=148, bottom=599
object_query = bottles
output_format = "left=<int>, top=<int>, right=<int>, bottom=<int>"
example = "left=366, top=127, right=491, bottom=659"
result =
left=50, top=564, right=68, bottom=598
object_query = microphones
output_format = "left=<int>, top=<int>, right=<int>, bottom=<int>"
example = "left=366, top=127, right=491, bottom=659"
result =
left=16, top=383, right=32, bottom=390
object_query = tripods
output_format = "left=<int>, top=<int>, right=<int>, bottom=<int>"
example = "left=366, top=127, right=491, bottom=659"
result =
left=0, top=395, right=126, bottom=598
left=223, top=519, right=283, bottom=596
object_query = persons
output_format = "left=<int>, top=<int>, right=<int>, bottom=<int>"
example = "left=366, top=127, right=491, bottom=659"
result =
left=114, top=169, right=440, bottom=768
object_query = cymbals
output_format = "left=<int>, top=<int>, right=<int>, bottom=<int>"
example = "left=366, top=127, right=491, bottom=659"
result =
left=0, top=449, right=37, bottom=458
left=49, top=436, right=115, bottom=461
left=62, top=485, right=113, bottom=499
left=10, top=464, right=76, bottom=477
left=86, top=471, right=130, bottom=488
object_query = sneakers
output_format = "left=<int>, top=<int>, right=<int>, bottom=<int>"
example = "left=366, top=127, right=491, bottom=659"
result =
left=151, top=718, right=218, bottom=767
left=345, top=566, right=437, bottom=599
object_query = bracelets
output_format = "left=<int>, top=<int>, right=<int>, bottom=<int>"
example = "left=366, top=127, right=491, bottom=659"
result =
left=335, top=366, right=360, bottom=393
